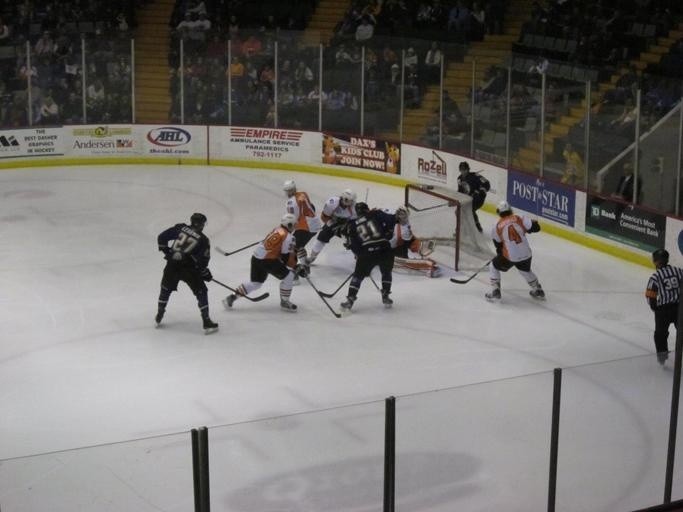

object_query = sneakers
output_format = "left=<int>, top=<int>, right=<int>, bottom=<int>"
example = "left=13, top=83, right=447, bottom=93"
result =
left=656, top=352, right=668, bottom=361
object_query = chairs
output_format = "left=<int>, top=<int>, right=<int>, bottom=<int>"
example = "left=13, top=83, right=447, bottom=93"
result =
left=0, top=0, right=682, bottom=203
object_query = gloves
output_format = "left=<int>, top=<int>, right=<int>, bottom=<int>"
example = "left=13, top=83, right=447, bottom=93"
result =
left=295, top=263, right=307, bottom=278
left=202, top=267, right=213, bottom=282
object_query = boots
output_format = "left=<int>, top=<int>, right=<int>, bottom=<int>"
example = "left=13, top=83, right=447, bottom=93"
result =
left=529, top=284, right=544, bottom=297
left=156, top=308, right=166, bottom=323
left=203, top=318, right=219, bottom=329
left=227, top=288, right=238, bottom=307
left=381, top=290, right=393, bottom=303
left=484, top=287, right=501, bottom=299
left=340, top=296, right=357, bottom=309
left=307, top=256, right=316, bottom=264
left=281, top=295, right=297, bottom=309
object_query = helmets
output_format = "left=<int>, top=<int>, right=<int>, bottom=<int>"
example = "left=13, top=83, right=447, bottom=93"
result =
left=459, top=162, right=469, bottom=170
left=355, top=202, right=369, bottom=217
left=280, top=213, right=299, bottom=233
left=282, top=179, right=296, bottom=198
left=340, top=188, right=357, bottom=207
left=396, top=206, right=410, bottom=220
left=190, top=213, right=208, bottom=227
left=495, top=201, right=511, bottom=217
left=651, top=249, right=669, bottom=267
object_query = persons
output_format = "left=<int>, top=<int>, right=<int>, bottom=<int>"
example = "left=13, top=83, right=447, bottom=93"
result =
left=221, top=214, right=300, bottom=309
left=388, top=208, right=421, bottom=258
left=283, top=181, right=316, bottom=285
left=307, top=189, right=357, bottom=263
left=453, top=162, right=490, bottom=233
left=611, top=164, right=643, bottom=204
left=169, top=0, right=683, bottom=184
left=484, top=201, right=547, bottom=298
left=341, top=201, right=401, bottom=308
left=646, top=250, right=683, bottom=353
left=155, top=213, right=220, bottom=329
left=0, top=1, right=133, bottom=123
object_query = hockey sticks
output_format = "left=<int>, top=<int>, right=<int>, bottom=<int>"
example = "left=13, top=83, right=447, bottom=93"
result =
left=306, top=275, right=352, bottom=318
left=449, top=259, right=492, bottom=284
left=211, top=277, right=269, bottom=302
left=369, top=274, right=391, bottom=295
left=215, top=240, right=263, bottom=256
left=408, top=202, right=449, bottom=212
